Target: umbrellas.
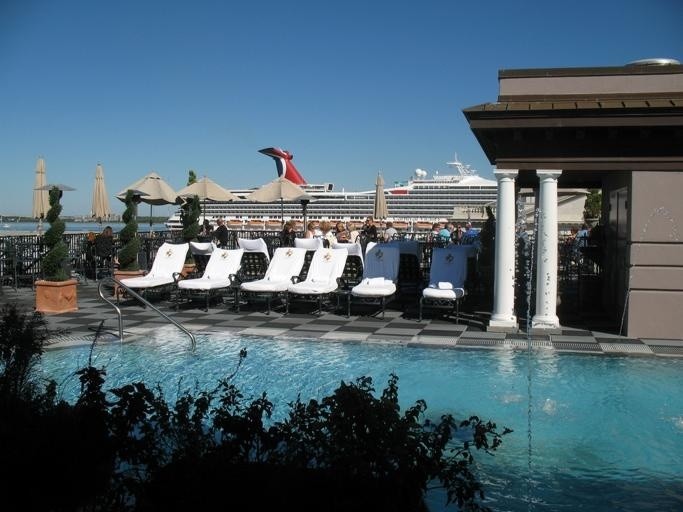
[91,159,112,233]
[114,168,389,234]
[32,153,51,235]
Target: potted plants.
[32,185,79,316]
[113,171,201,307]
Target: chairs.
[391,240,429,292]
[238,238,269,282]
[294,238,323,281]
[448,244,476,265]
[419,246,469,325]
[285,248,348,317]
[178,248,244,312]
[190,242,217,278]
[332,243,365,314]
[239,247,308,315]
[120,241,189,309]
[347,242,401,323]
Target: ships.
[164,145,499,236]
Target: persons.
[96,225,113,258]
[429,222,478,241]
[280,216,399,246]
[197,219,228,248]
